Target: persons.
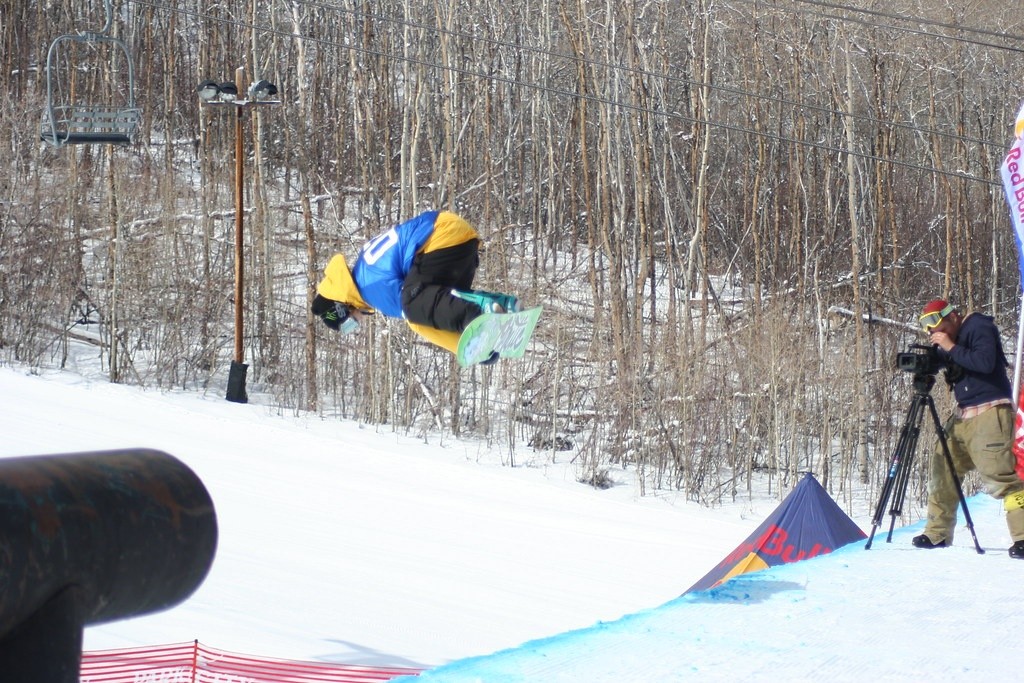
[310,209,519,367]
[911,299,1024,560]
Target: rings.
[938,333,940,336]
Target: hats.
[311,294,349,334]
[924,297,947,313]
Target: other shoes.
[479,302,504,365]
[1009,541,1024,558]
[913,535,945,548]
[506,297,525,313]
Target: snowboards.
[456,305,544,367]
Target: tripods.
[864,375,984,554]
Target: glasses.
[338,315,360,335]
[919,311,942,332]
